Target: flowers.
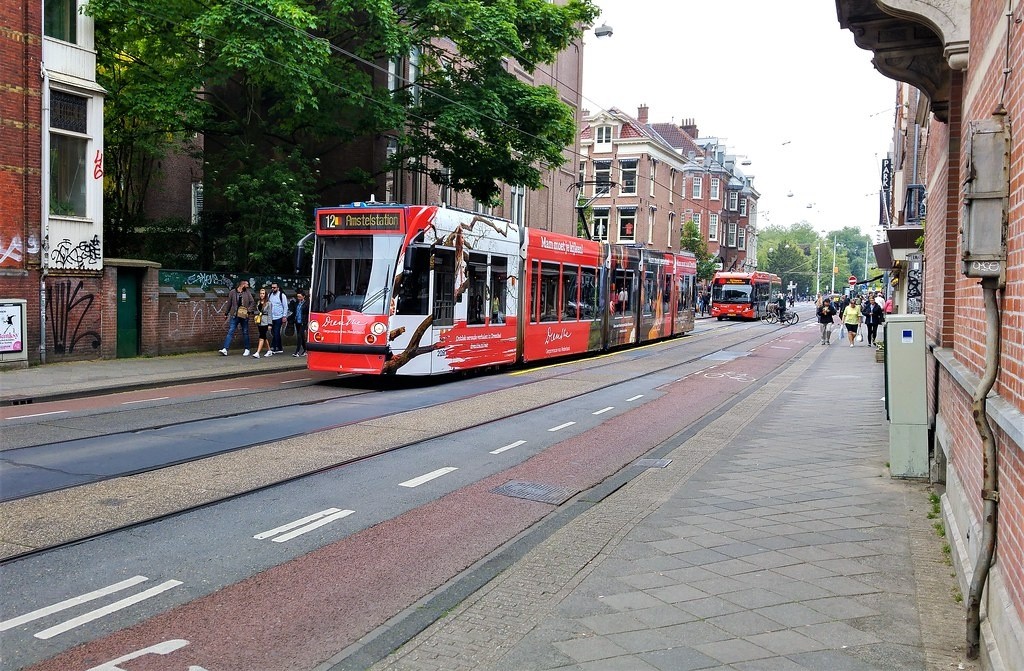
[873,339,884,350]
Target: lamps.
[821,228,826,233]
[785,243,790,248]
[805,203,813,208]
[786,192,794,197]
[740,158,752,165]
[835,242,842,247]
[768,244,774,252]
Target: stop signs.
[848,276,857,286]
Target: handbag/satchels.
[254,312,262,324]
[839,325,844,339]
[856,327,863,342]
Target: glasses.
[851,303,855,304]
[271,287,276,289]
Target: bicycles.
[760,304,800,326]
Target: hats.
[267,330,274,339]
[877,293,881,295]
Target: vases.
[875,348,884,363]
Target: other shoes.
[868,343,871,347]
[872,339,876,346]
[827,341,830,345]
[822,341,825,345]
[292,352,300,357]
[219,348,227,356]
[243,349,251,356]
[850,344,855,348]
[264,351,272,357]
[272,350,283,354]
[301,350,308,356]
[253,352,260,358]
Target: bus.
[708,271,783,321]
[304,181,699,378]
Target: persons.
[771,293,786,325]
[267,280,288,354]
[610,287,629,316]
[698,290,711,313]
[817,298,837,346]
[290,290,309,357]
[862,298,884,347]
[252,287,274,358]
[813,290,893,325]
[218,278,256,357]
[474,291,483,323]
[842,298,863,348]
[777,291,815,308]
[493,293,500,323]
